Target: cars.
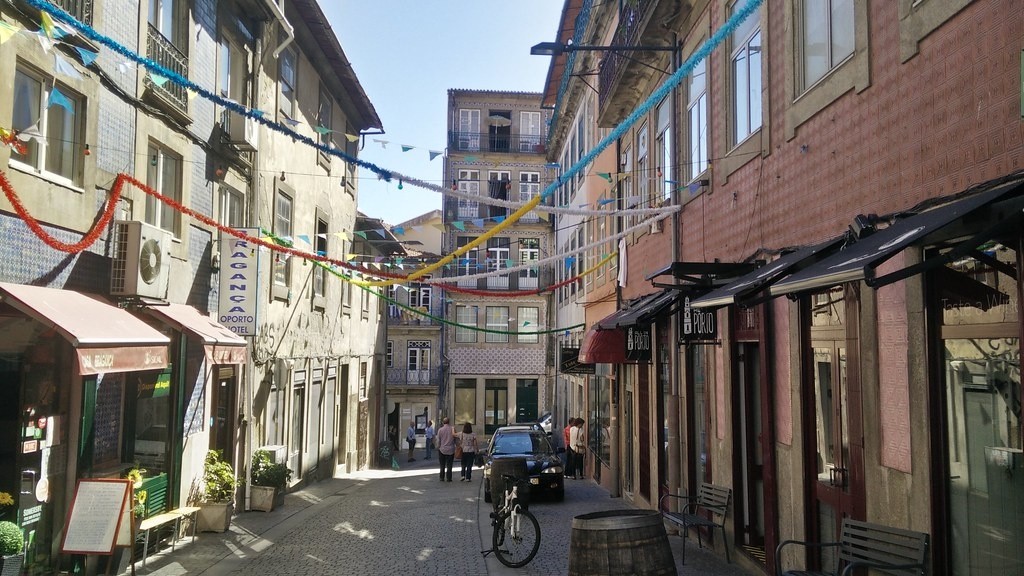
[507,412,552,437]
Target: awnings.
[0,283,171,377]
[688,234,856,309]
[645,260,767,299]
[140,301,249,365]
[590,290,710,333]
[578,308,660,364]
[761,170,1024,303]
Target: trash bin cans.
[378,440,393,468]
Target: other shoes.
[440,478,444,481]
[460,477,465,481]
[466,479,469,481]
[571,477,576,480]
[580,477,585,479]
[447,480,452,482]
[408,460,413,462]
[411,458,416,461]
[567,476,574,478]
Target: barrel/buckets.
[568,509,677,576]
[489,458,529,511]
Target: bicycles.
[480,472,541,569]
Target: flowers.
[0,491,14,508]
[123,459,148,519]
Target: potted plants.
[196,448,244,533]
[0,520,25,576]
[249,448,295,513]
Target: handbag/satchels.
[454,446,463,459]
[406,436,412,442]
[431,442,435,449]
[576,443,586,459]
[475,453,484,467]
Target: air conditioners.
[109,217,175,299]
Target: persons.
[406,421,416,462]
[425,421,434,460]
[564,417,576,478]
[452,422,479,482]
[569,418,586,480]
[389,423,399,451]
[437,418,459,482]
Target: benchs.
[775,510,930,576]
[659,481,731,564]
[92,457,136,478]
[138,506,201,567]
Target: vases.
[98,516,142,576]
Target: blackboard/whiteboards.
[58,477,134,555]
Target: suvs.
[482,426,565,502]
